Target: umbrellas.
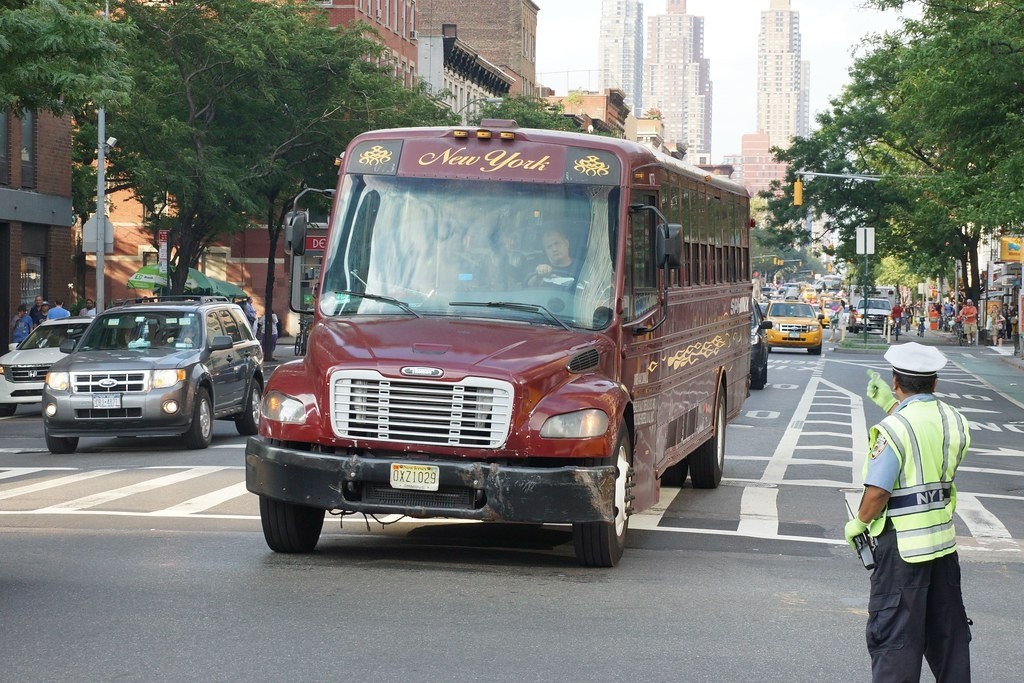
[126,263,249,300]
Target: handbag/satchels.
[997,323,1003,330]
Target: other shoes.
[967,341,975,347]
[991,343,997,346]
[838,340,845,342]
[827,339,834,343]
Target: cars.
[750,282,851,390]
[0,315,190,417]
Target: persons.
[258,310,278,361]
[890,299,1019,347]
[167,325,196,349]
[829,301,846,342]
[245,296,256,329]
[845,342,972,683]
[524,225,584,291]
[78,299,97,317]
[10,296,70,348]
[117,325,132,350]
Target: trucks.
[849,285,896,335]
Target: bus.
[245,119,756,569]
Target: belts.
[883,519,896,532]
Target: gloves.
[867,369,898,413]
[845,511,868,550]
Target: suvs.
[42,295,265,454]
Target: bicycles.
[294,319,307,356]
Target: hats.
[967,299,972,302]
[40,301,49,305]
[884,342,948,376]
